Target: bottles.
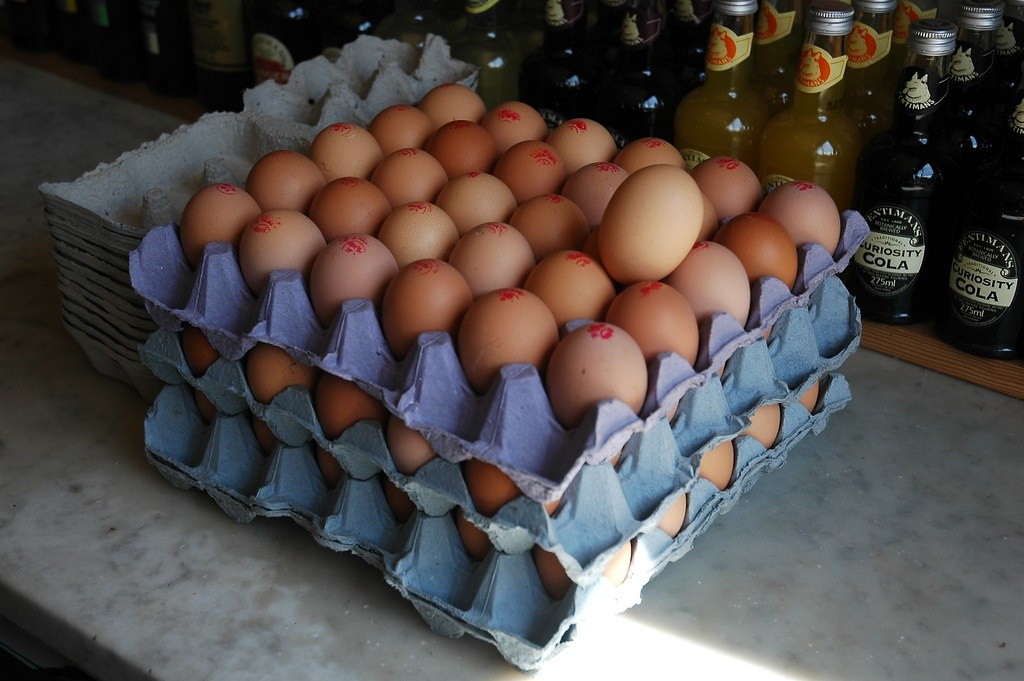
[892,0,939,69]
[844,0,899,142]
[852,19,959,326]
[751,0,805,120]
[0,0,713,184]
[758,0,861,220]
[676,0,769,171]
[931,0,1024,359]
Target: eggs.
[177,82,840,594]
